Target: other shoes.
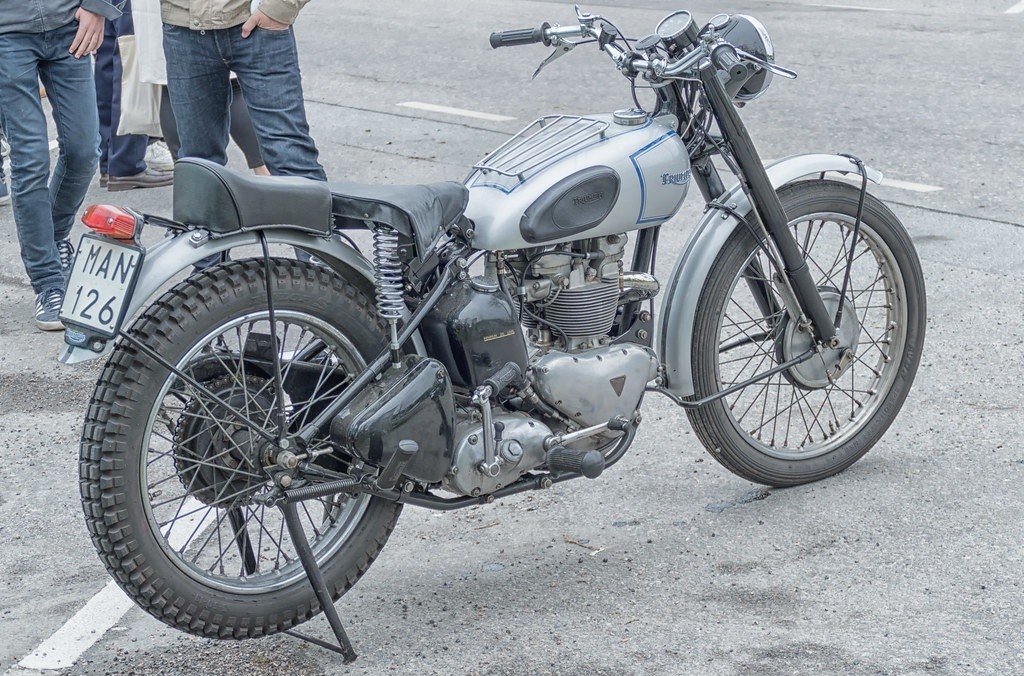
[0,178,10,203]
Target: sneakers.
[55,236,74,276]
[34,287,65,330]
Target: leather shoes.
[107,167,173,191]
[99,174,108,186]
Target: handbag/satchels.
[116,34,165,138]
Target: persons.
[0,0,127,332]
[131,0,340,278]
[94,0,174,192]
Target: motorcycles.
[58,3,928,662]
